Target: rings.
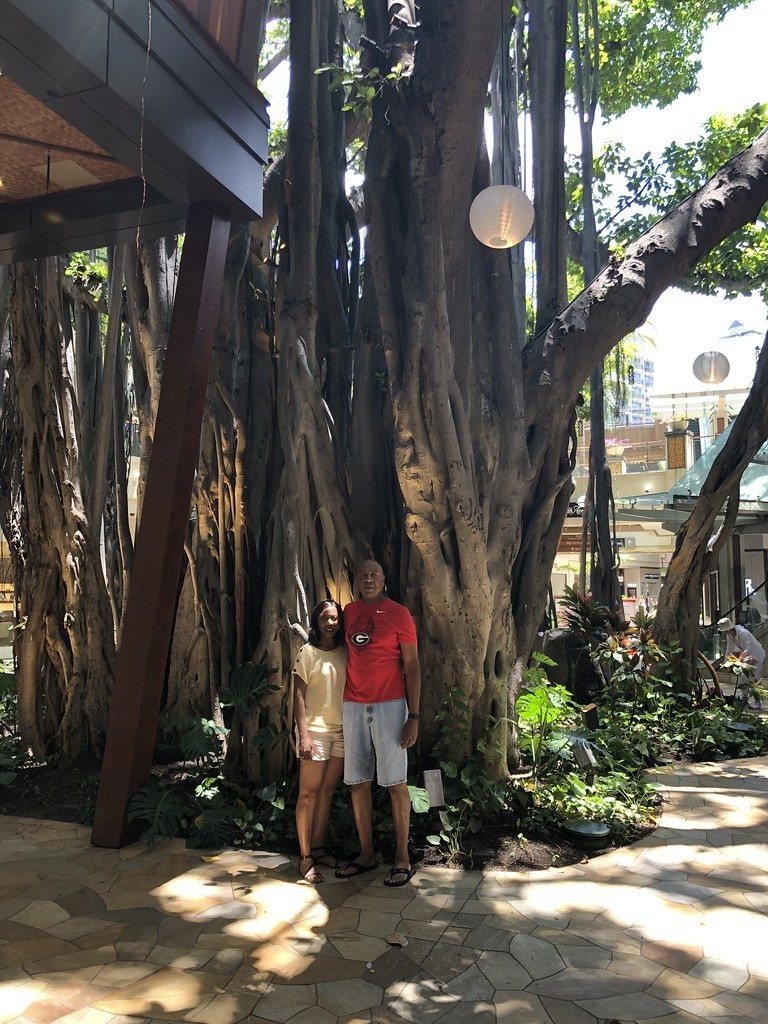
[412,742,416,744]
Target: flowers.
[605,437,632,448]
[659,413,697,426]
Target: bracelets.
[408,714,419,720]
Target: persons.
[335,560,420,886]
[291,599,349,884]
[717,619,766,709]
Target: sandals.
[311,847,349,869]
[299,854,324,883]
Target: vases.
[607,447,625,458]
[671,420,690,430]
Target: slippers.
[384,864,416,887]
[335,858,378,878]
